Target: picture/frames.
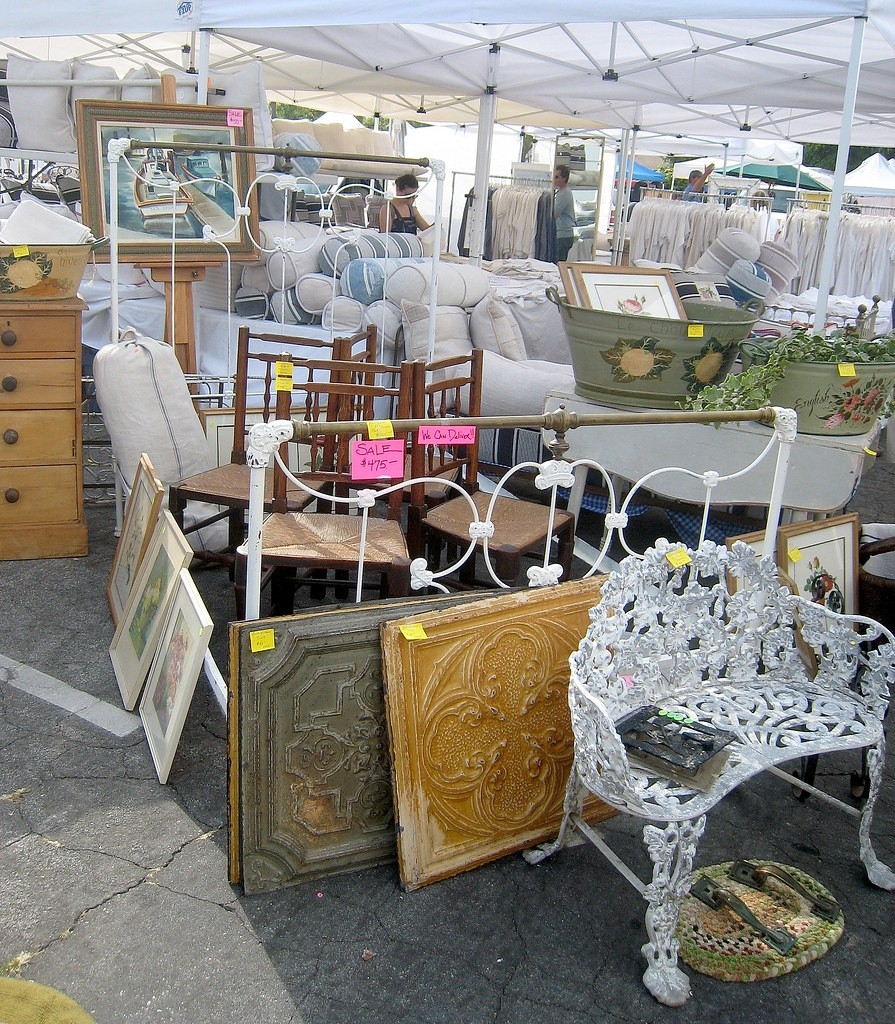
[139,567,214,785]
[571,265,688,321]
[725,520,814,709]
[75,98,262,264]
[107,452,165,629]
[777,511,860,671]
[108,509,194,711]
[557,261,656,307]
[200,405,371,529]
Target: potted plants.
[674,323,895,436]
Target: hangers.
[791,199,895,223]
[730,195,769,213]
[489,174,551,193]
[643,187,727,211]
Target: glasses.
[555,175,561,179]
[401,190,418,199]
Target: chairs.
[335,323,462,602]
[412,347,577,596]
[522,537,895,1008]
[234,350,414,619]
[169,325,342,624]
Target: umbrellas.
[716,162,833,191]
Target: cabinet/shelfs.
[0,290,90,561]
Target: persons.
[379,175,430,236]
[553,165,576,262]
[685,163,715,205]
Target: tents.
[615,154,760,191]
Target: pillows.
[266,229,378,291]
[567,170,582,187]
[276,118,395,226]
[321,295,366,332]
[755,240,800,308]
[0,53,275,217]
[662,267,737,310]
[235,288,272,320]
[240,264,275,296]
[270,291,321,326]
[236,222,326,266]
[400,299,474,413]
[382,264,489,308]
[317,233,424,279]
[725,260,772,313]
[684,227,760,274]
[631,259,682,272]
[296,273,341,315]
[469,291,528,361]
[453,349,578,429]
[339,257,438,305]
[363,300,406,349]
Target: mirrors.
[552,135,606,261]
